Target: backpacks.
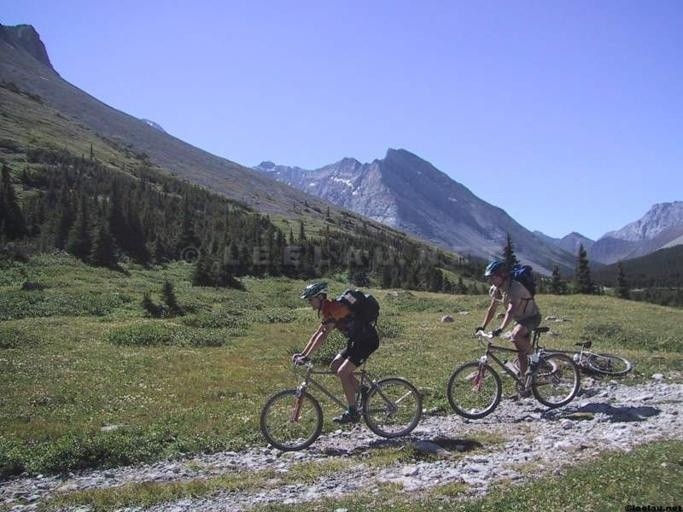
[333,287,379,324]
[507,264,535,301]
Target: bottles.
[573,354,578,362]
[502,358,520,376]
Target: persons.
[472,260,542,401]
[291,280,380,424]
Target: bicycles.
[445,324,583,420]
[510,340,634,377]
[257,354,424,454]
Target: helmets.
[300,282,327,300]
[483,259,505,278]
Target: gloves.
[292,354,305,363]
[492,328,501,336]
[295,356,307,366]
[476,326,483,332]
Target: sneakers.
[360,386,370,407]
[509,390,532,399]
[524,354,540,376]
[331,411,360,424]
